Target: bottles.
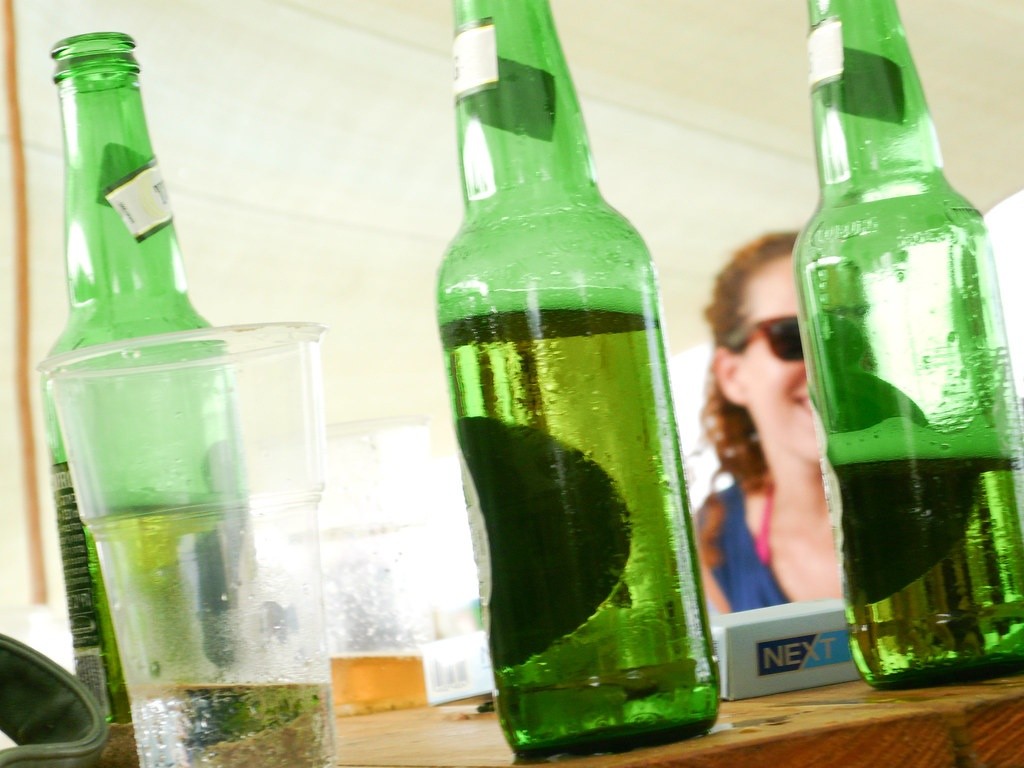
[790,2,1023,696]
[38,32,288,768]
[434,1,721,764]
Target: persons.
[695,231,844,614]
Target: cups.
[40,321,335,766]
[314,412,443,719]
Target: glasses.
[728,316,806,362]
